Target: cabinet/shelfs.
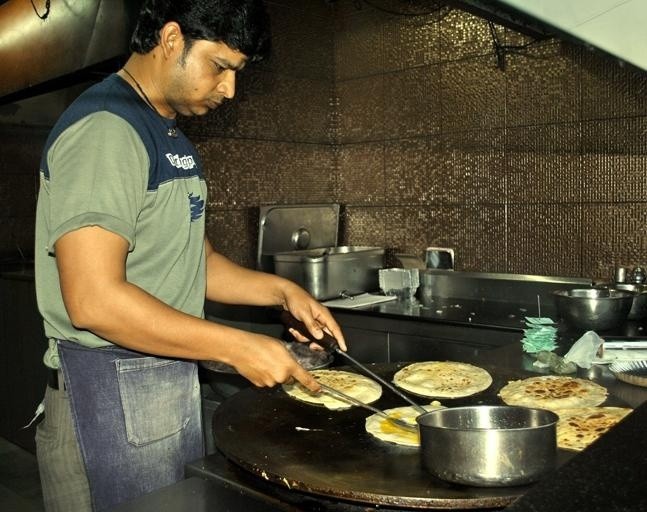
[328,289,647,364]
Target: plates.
[608,360,647,388]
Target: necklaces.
[122,67,178,138]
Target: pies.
[547,408,606,450]
[280,369,381,411]
[394,361,493,399]
[365,400,448,447]
[499,375,607,408]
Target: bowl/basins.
[199,342,334,399]
[554,288,640,330]
[594,283,647,318]
[415,405,559,487]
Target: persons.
[22,0,347,512]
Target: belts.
[47,366,66,391]
